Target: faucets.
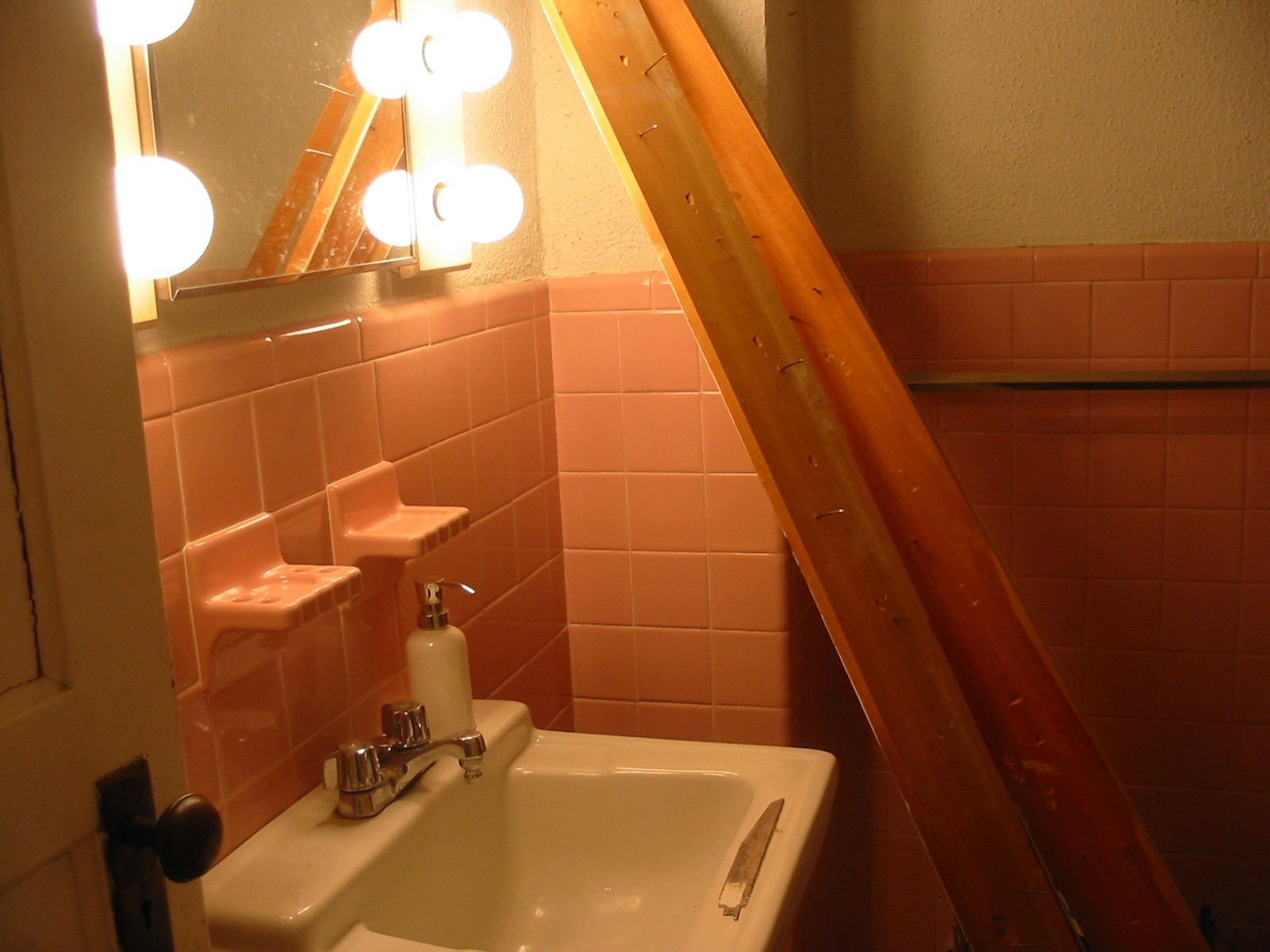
[337,698,487,823]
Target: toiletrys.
[405,574,479,738]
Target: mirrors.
[130,0,418,302]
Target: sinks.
[201,697,845,951]
